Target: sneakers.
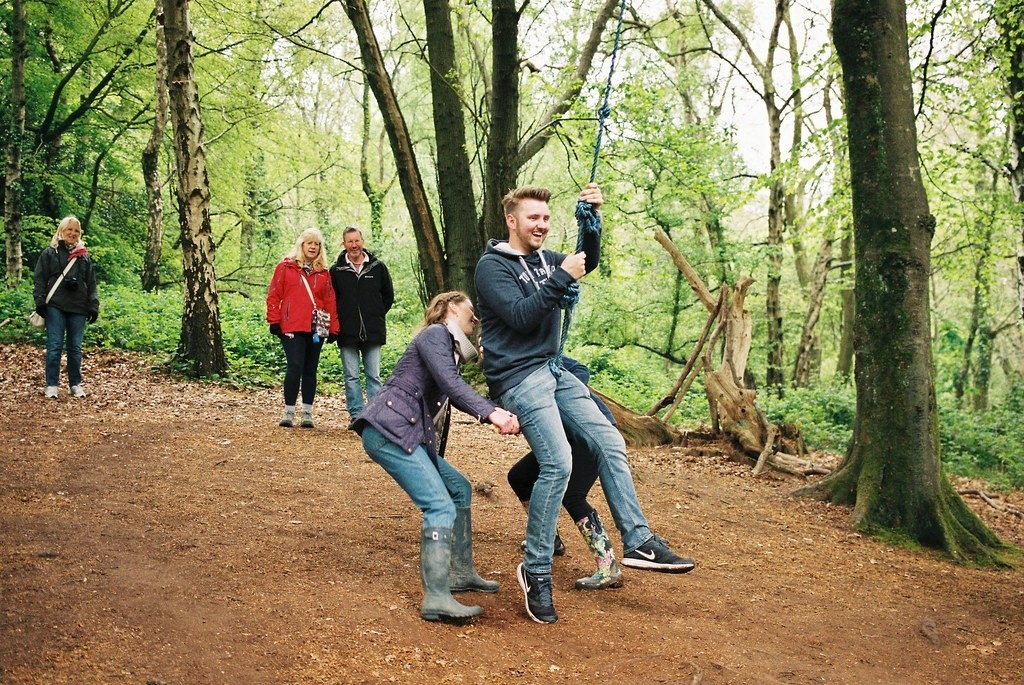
[620,533,695,574]
[517,562,559,624]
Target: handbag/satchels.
[311,308,330,336]
[29,310,45,329]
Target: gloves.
[36,304,47,317]
[270,322,281,334]
[326,333,337,343]
[70,384,86,397]
[87,310,98,323]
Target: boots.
[521,500,566,555]
[420,526,483,620]
[448,507,499,592]
[575,508,623,590]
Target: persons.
[329,226,394,431]
[348,291,515,624]
[477,326,626,591]
[32,216,101,400]
[473,181,695,624]
[267,227,340,429]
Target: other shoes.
[300,411,314,427]
[45,385,58,398]
[279,410,295,426]
